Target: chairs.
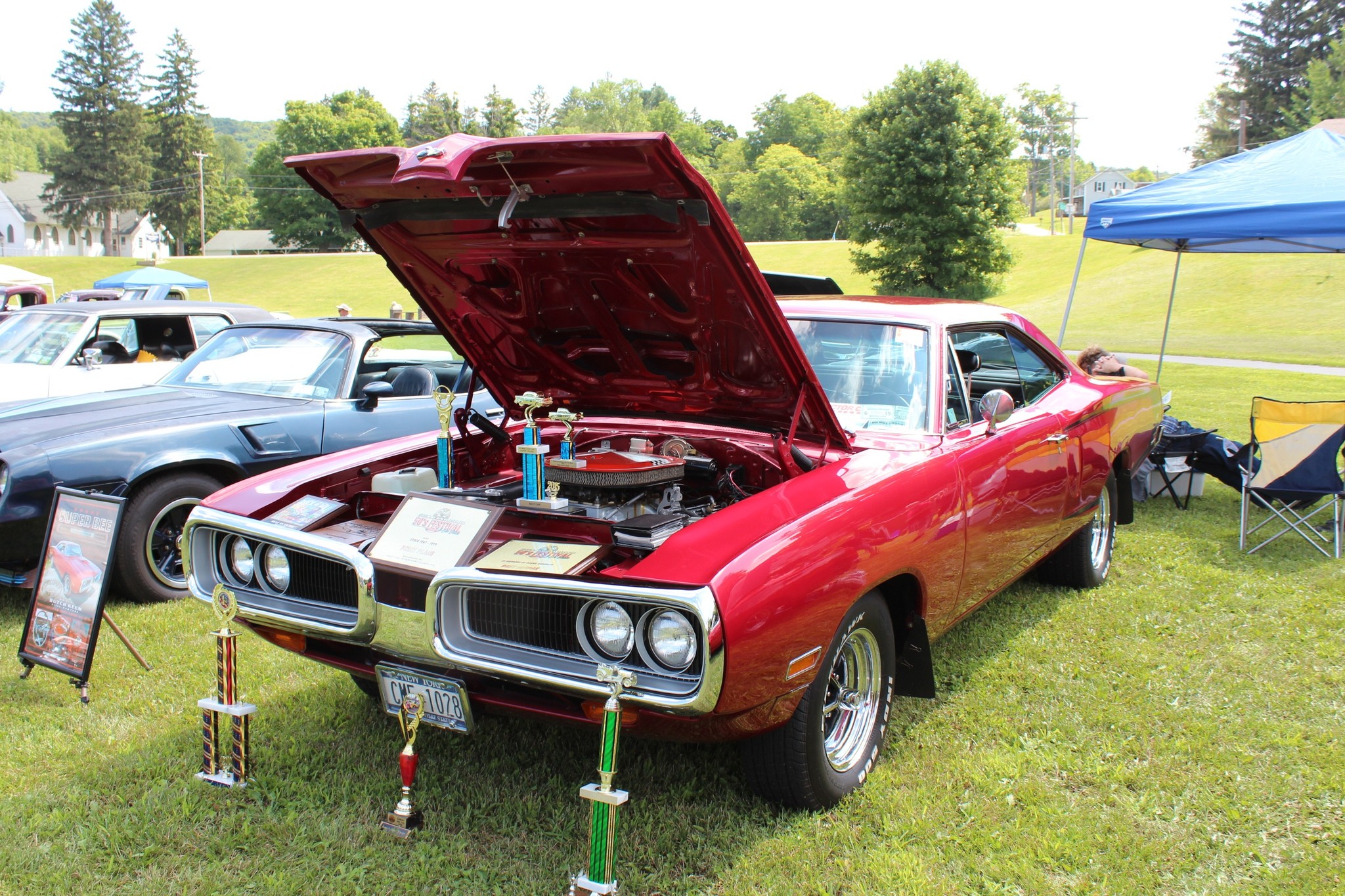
[947,348,986,424]
[389,366,440,400]
[1148,406,1219,511]
[135,337,180,363]
[92,340,130,365]
[1223,397,1345,558]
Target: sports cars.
[0,318,505,602]
[0,301,452,430]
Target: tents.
[1058,126,1345,382]
[0,264,213,304]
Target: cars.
[0,284,189,323]
[181,132,1164,896]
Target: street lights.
[1062,103,1088,235]
[1038,122,1065,235]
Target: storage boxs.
[1146,464,1206,496]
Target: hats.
[337,304,352,311]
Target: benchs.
[358,368,482,396]
[823,373,1039,410]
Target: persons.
[1075,346,1323,512]
[337,303,352,317]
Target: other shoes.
[1286,497,1322,509]
